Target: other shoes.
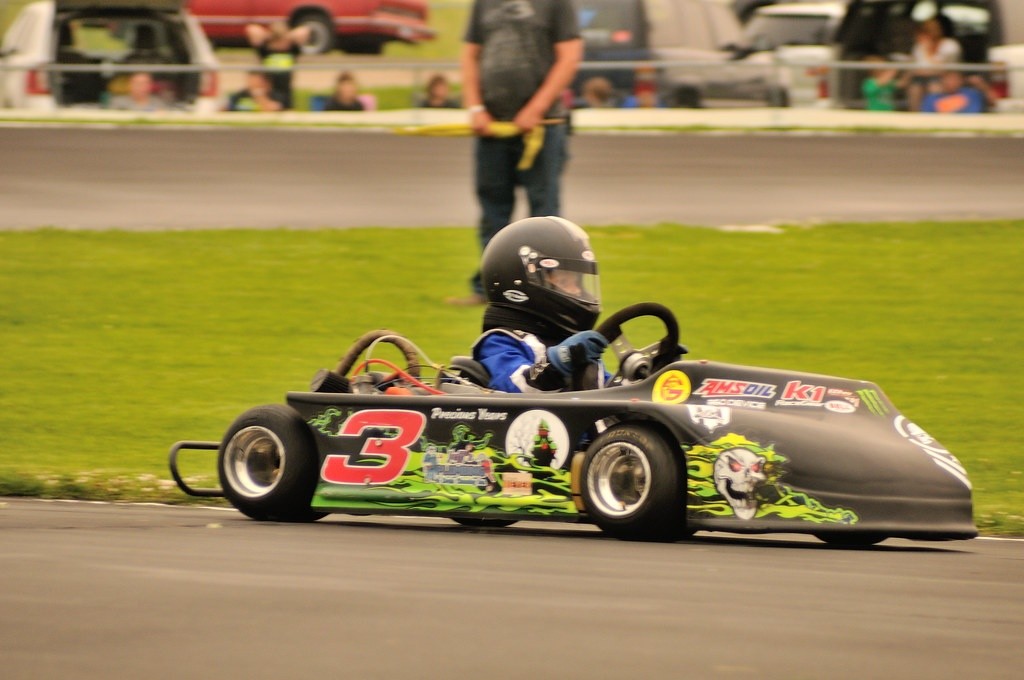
[444,294,485,307]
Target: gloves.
[652,344,688,373]
[547,330,608,377]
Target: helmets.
[480,215,600,334]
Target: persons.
[109,23,704,109]
[862,15,984,113]
[449,0,584,307]
[472,216,688,393]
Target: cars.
[558,0,1023,119]
[185,0,437,57]
[1,0,232,121]
